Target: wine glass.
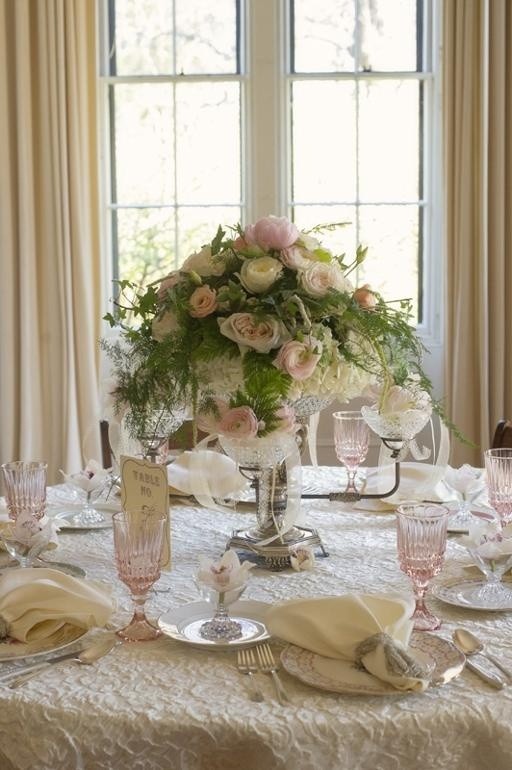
[332,412,371,493]
[484,448,511,541]
[467,545,511,599]
[441,476,487,521]
[64,474,108,520]
[0,526,49,568]
[395,503,447,629]
[193,579,247,637]
[1,460,47,522]
[112,513,166,643]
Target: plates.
[45,503,120,529]
[1,562,86,582]
[431,576,511,612]
[156,597,276,651]
[446,509,496,534]
[0,621,90,662]
[281,629,464,695]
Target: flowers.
[96,214,478,503]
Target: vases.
[124,392,434,571]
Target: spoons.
[455,628,512,685]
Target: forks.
[10,637,116,689]
[255,644,288,707]
[234,648,265,704]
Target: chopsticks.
[1,641,124,680]
